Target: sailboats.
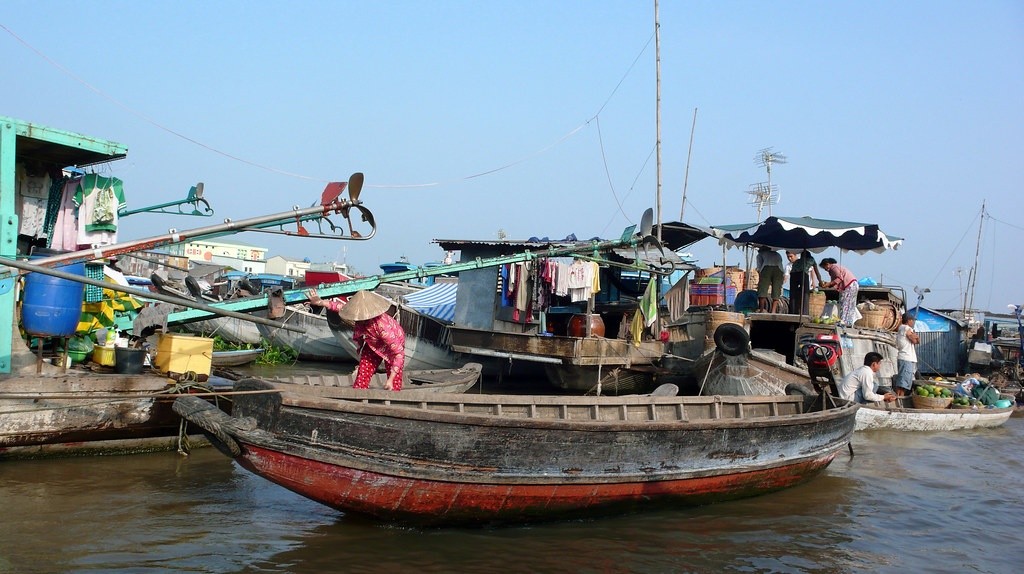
[168,0,861,521]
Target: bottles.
[105,326,115,347]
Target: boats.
[1,117,486,450]
[677,149,1024,435]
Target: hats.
[902,313,917,321]
[758,246,771,253]
[338,291,391,321]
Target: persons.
[304,289,405,391]
[784,251,816,297]
[839,352,896,405]
[977,323,985,342]
[790,250,823,314]
[895,313,920,397]
[756,246,783,313]
[820,258,859,325]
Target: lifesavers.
[171,394,257,459]
[185,276,202,298]
[714,322,750,358]
[241,280,259,295]
[152,274,164,290]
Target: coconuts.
[915,385,982,406]
[813,315,840,325]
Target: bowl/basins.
[56,349,88,361]
[996,399,1011,408]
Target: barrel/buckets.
[56,353,72,369]
[22,247,85,338]
[114,344,148,374]
[43,358,51,362]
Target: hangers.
[80,162,115,181]
[575,247,594,263]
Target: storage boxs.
[94,344,117,366]
[688,269,736,306]
[155,333,214,377]
